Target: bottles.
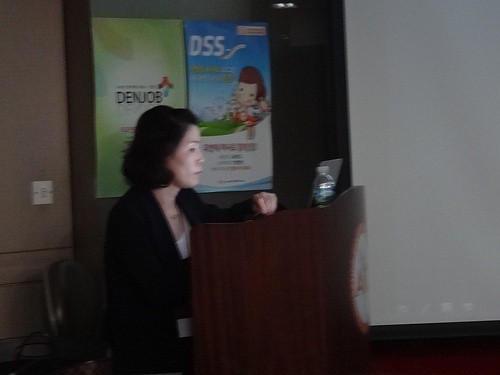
[312,166,337,208]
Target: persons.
[98,105,277,375]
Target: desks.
[0,336,113,375]
